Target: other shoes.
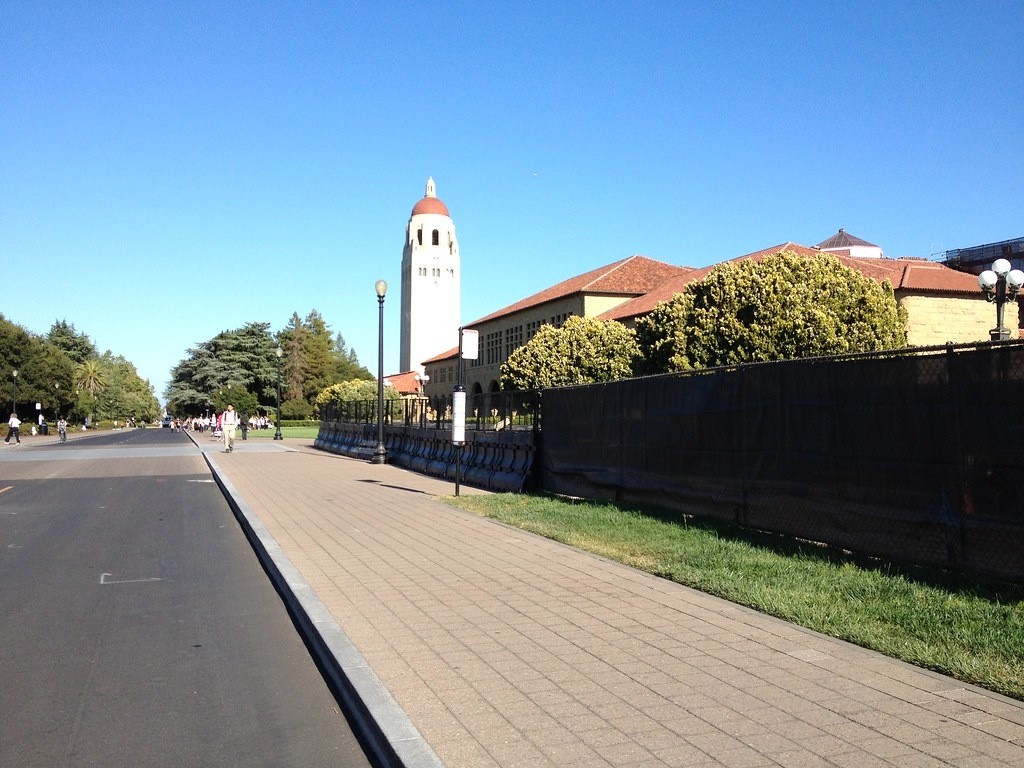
[17,442,19,444]
[243,437,247,440]
[226,449,229,453]
[230,447,233,451]
[4,441,10,445]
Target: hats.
[243,412,246,414]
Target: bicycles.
[58,427,66,445]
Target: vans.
[162,415,173,428]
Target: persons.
[141,421,145,428]
[58,418,67,441]
[175,419,181,432]
[240,412,249,440]
[114,420,117,429]
[3,413,21,445]
[183,417,210,433]
[218,412,223,431]
[220,403,238,452]
[249,416,274,430]
[210,412,218,436]
[169,420,176,433]
[120,422,124,429]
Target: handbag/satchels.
[213,427,221,437]
[240,421,246,425]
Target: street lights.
[12,370,18,415]
[219,391,223,411]
[974,259,1023,340]
[54,383,61,419]
[273,345,284,440]
[226,383,232,407]
[370,279,388,465]
[75,389,80,428]
[412,374,430,399]
